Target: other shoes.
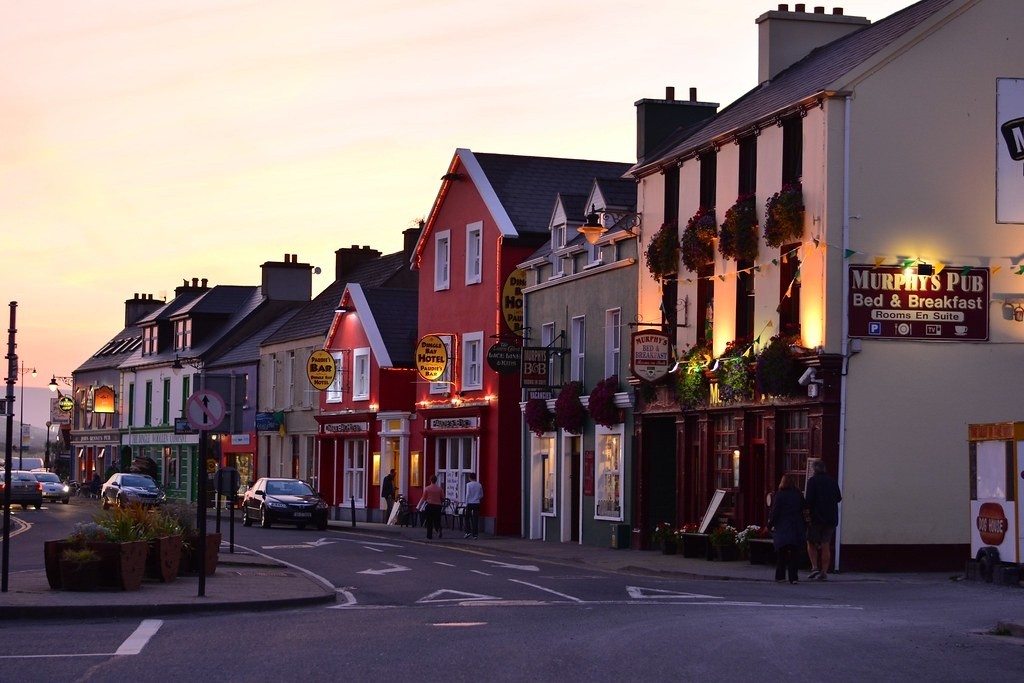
[438,528,442,538]
[425,538,430,542]
[775,578,786,583]
[808,569,820,578]
[473,536,477,540]
[791,580,798,585]
[818,572,826,579]
[464,533,471,539]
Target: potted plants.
[121,494,222,590]
[59,547,102,592]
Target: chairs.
[398,496,466,531]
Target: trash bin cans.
[610,523,630,549]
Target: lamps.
[48,374,76,393]
[169,354,203,376]
[669,357,732,372]
[577,203,641,245]
[917,257,932,275]
[334,306,350,313]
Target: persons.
[463,473,484,540]
[86,461,129,498]
[767,475,809,584]
[805,460,842,580]
[381,469,400,524]
[422,476,446,540]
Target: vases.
[659,540,678,555]
[679,531,708,557]
[748,537,775,564]
[45,539,120,592]
[716,543,741,562]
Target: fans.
[652,522,700,544]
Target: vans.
[11,456,44,472]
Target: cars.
[29,472,71,504]
[0,470,43,510]
[241,477,330,531]
[0,458,8,471]
[100,473,167,512]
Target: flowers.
[716,331,754,402]
[525,399,552,437]
[718,193,758,263]
[673,337,713,417]
[554,380,586,434]
[643,219,680,284]
[588,374,619,431]
[755,332,801,402]
[679,206,717,273]
[762,184,803,249]
[709,524,773,553]
[63,502,144,544]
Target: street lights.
[19,360,38,470]
